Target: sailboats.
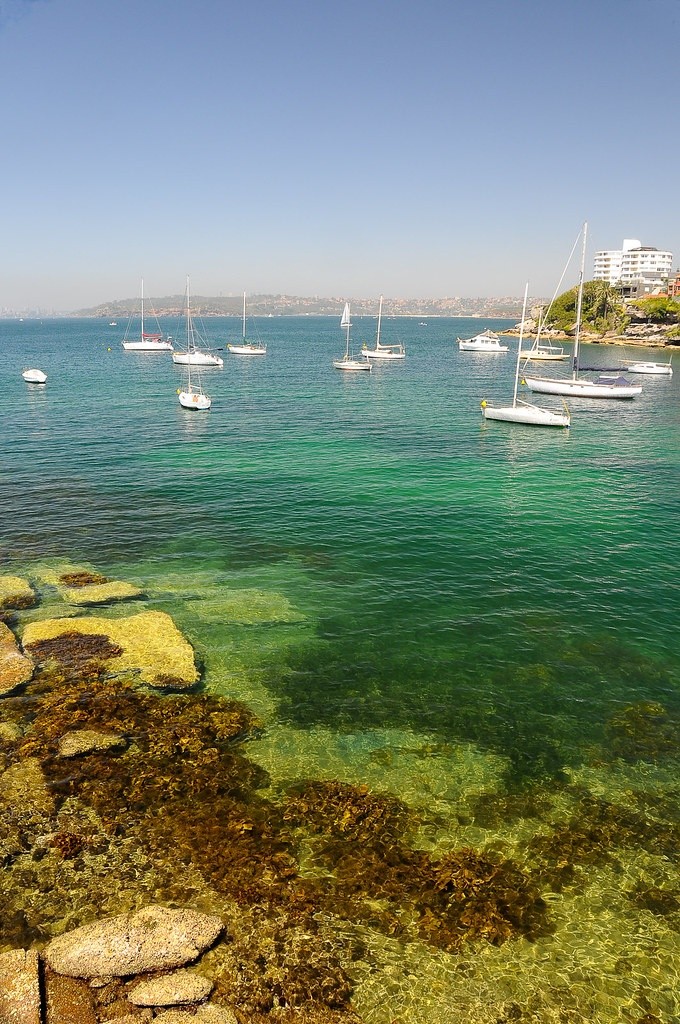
[517,309,571,360]
[480,280,572,428]
[120,275,267,411]
[331,294,406,371]
[513,220,645,399]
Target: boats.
[22,368,48,383]
[455,328,511,352]
[627,354,675,375]
